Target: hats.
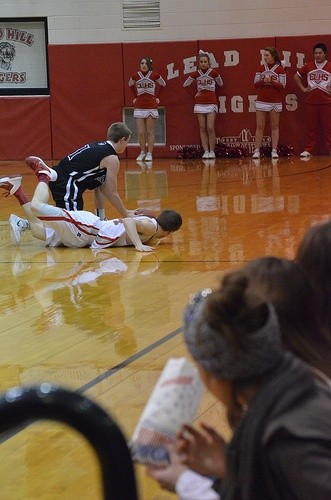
[181,279,281,377]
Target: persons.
[128,56,166,161]
[0,156,183,251]
[145,219,331,500]
[293,43,331,157]
[182,49,224,158]
[251,47,286,158]
[48,122,137,220]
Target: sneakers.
[300,150,311,157]
[0,174,22,198]
[24,155,59,181]
[8,214,30,245]
[252,149,260,159]
[271,150,279,159]
[201,151,216,160]
[134,152,154,161]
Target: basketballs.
[135,209,155,218]
